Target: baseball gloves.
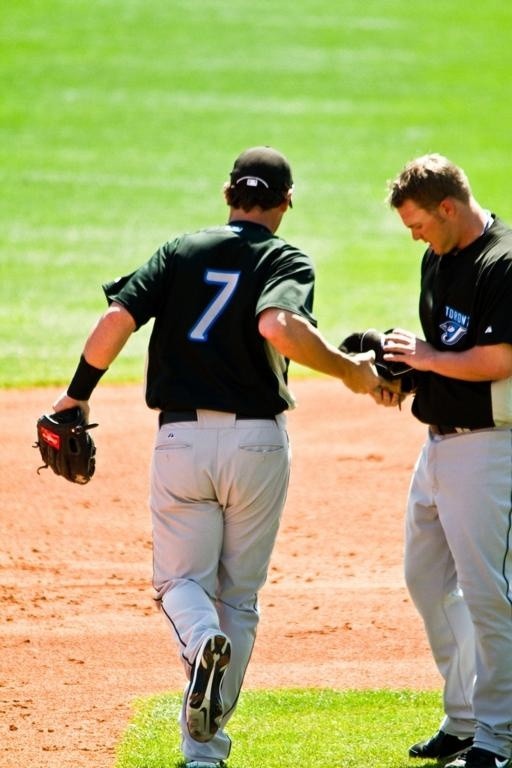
[31,406,98,484]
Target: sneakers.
[409,732,474,758]
[445,745,507,767]
[186,635,230,768]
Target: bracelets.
[65,357,106,402]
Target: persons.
[374,152,512,768]
[55,147,383,768]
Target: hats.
[229,145,294,209]
[338,329,422,393]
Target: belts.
[157,407,276,421]
[427,423,486,435]
